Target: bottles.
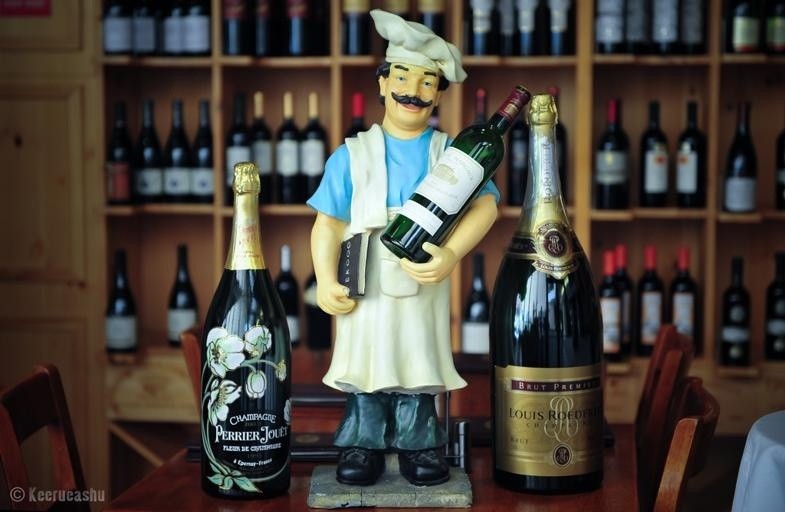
[593,98,785,212]
[466,0,577,56]
[343,93,368,144]
[106,88,331,204]
[489,92,608,497]
[273,244,303,348]
[598,242,785,369]
[196,160,294,502]
[340,0,446,57]
[724,1,785,53]
[595,1,707,55]
[104,1,332,57]
[163,241,203,346]
[377,75,531,266]
[106,242,138,365]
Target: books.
[335,231,372,299]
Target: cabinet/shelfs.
[103,0,785,512]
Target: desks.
[99,352,640,512]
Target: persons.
[305,6,500,487]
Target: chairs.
[180,323,205,413]
[634,322,719,512]
[0,361,93,512]
